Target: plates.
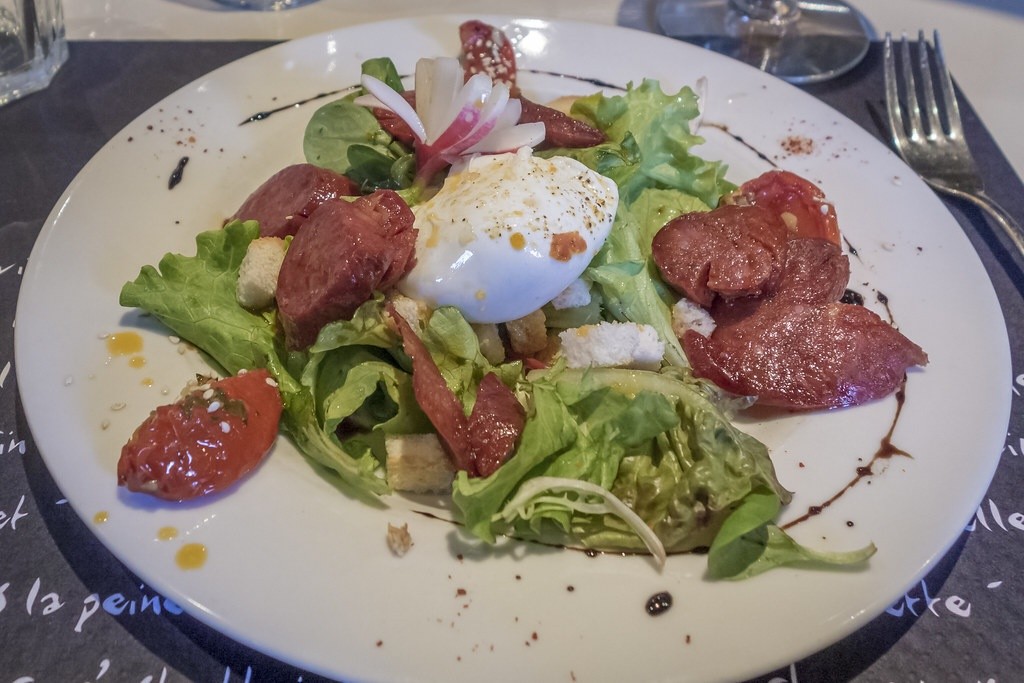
[14,15,1012,683]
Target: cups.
[0,0,69,109]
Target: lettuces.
[115,57,877,581]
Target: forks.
[884,27,1024,259]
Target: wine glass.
[654,0,871,84]
